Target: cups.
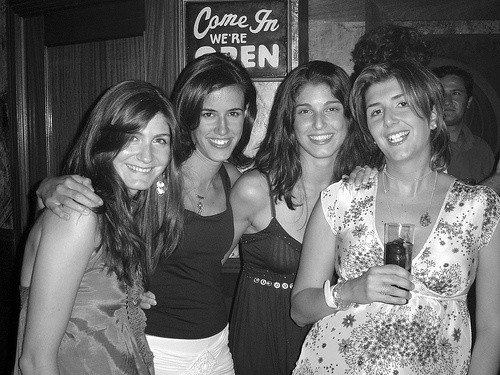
[384,222,415,305]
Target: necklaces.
[383,165,438,228]
[179,171,220,215]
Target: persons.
[36,52,258,375]
[290,57,500,375]
[0,79,185,375]
[220,61,380,375]
[434,67,497,185]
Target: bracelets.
[324,279,353,312]
